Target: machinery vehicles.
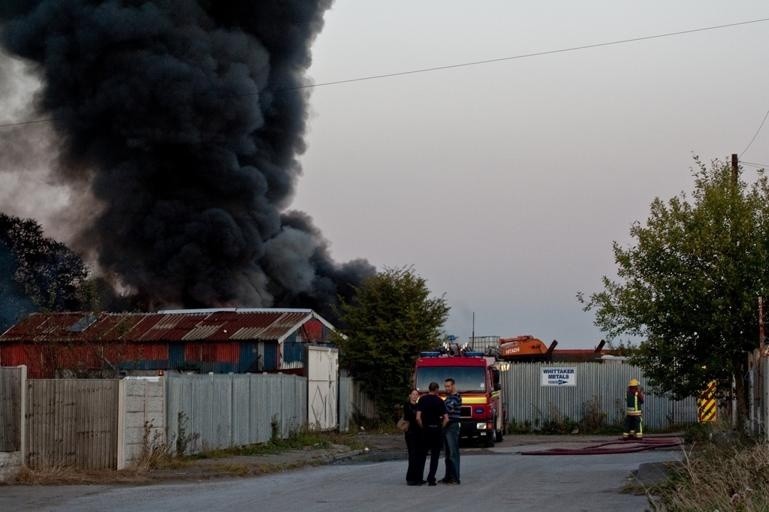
[481,334,549,354]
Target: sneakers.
[416,475,462,486]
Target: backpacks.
[397,418,410,433]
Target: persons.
[402,387,429,485]
[437,378,463,486]
[621,378,647,440]
[414,382,450,486]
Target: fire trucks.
[411,342,505,448]
